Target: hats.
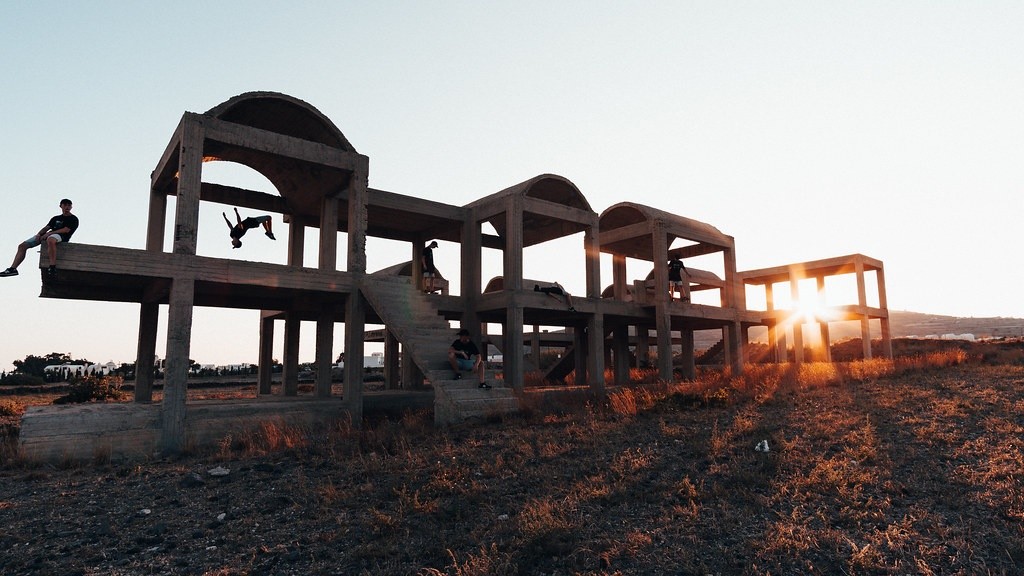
[534,285,539,291]
[432,241,438,248]
[61,199,72,205]
[457,329,469,336]
[233,242,241,248]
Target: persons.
[533,284,576,311]
[0,199,79,277]
[448,329,491,390]
[668,252,692,302]
[420,241,439,293]
[223,207,277,249]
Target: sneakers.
[0,268,18,277]
[48,268,58,281]
[569,306,577,313]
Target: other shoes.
[453,374,463,380]
[265,232,276,240]
[430,291,438,294]
[478,384,492,390]
